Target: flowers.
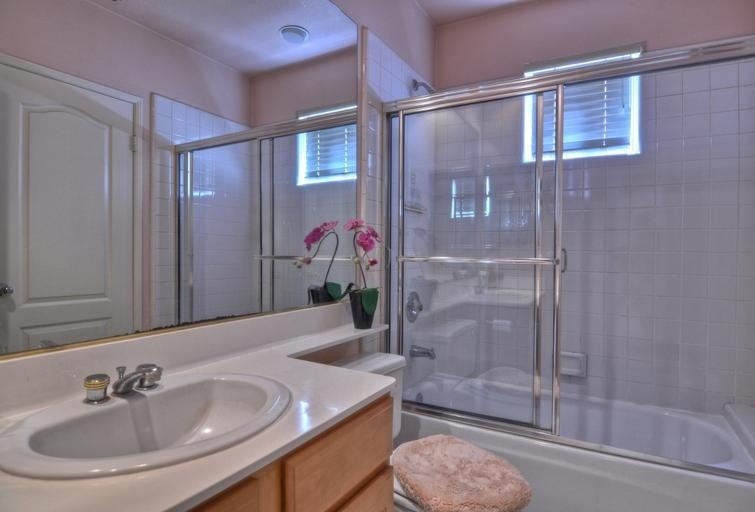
[292,219,342,300]
[344,215,383,315]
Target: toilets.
[335,353,518,510]
[430,320,549,390]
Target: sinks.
[1,370,292,477]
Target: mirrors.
[0,0,370,359]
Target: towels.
[391,432,533,512]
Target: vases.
[348,290,378,328]
[310,288,336,304]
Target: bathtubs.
[403,365,751,474]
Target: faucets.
[410,342,436,362]
[111,370,160,396]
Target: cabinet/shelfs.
[189,391,395,512]
